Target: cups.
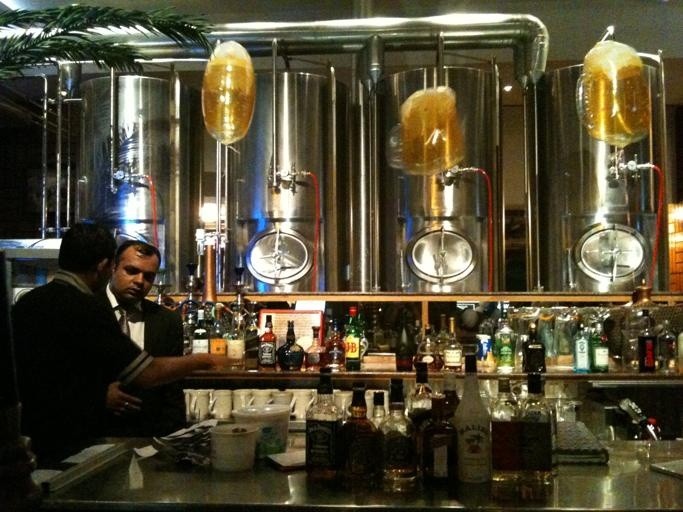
[179,386,355,420]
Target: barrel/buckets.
[232,402,289,456]
[211,423,258,471]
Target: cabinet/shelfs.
[141,287,682,386]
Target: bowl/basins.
[209,405,288,473]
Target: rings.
[121,400,129,408]
[119,406,125,411]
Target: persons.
[97,240,188,439]
[8,221,232,472]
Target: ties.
[118,308,135,338]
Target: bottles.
[304,361,582,502]
[186,302,678,375]
